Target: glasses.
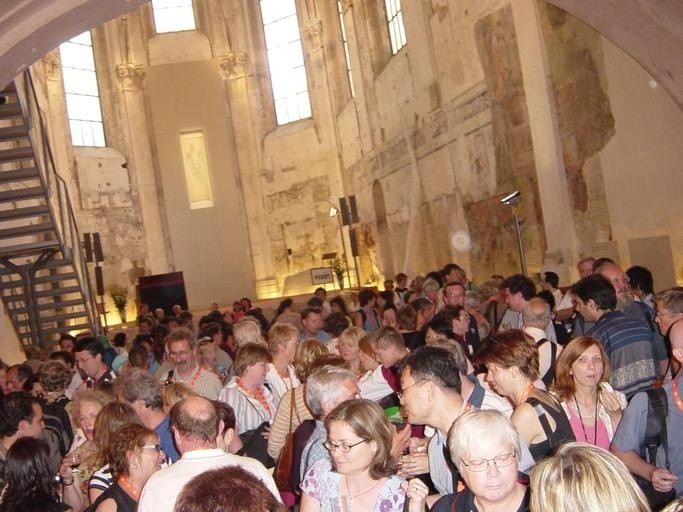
[322,438,372,455]
[168,350,193,360]
[140,444,163,454]
[395,378,431,401]
[460,447,519,473]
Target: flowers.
[328,257,346,279]
[110,288,131,310]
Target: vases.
[338,280,343,289]
[119,310,128,328]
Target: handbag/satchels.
[274,432,292,493]
[237,421,277,470]
[634,475,678,512]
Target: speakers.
[93,233,104,261]
[349,196,358,223]
[95,266,104,295]
[84,233,93,261]
[349,229,358,256]
[339,198,350,226]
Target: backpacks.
[37,394,75,477]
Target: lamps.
[330,207,351,289]
[501,190,528,279]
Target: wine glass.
[69,453,81,474]
[387,408,409,435]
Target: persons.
[1,258,682,512]
